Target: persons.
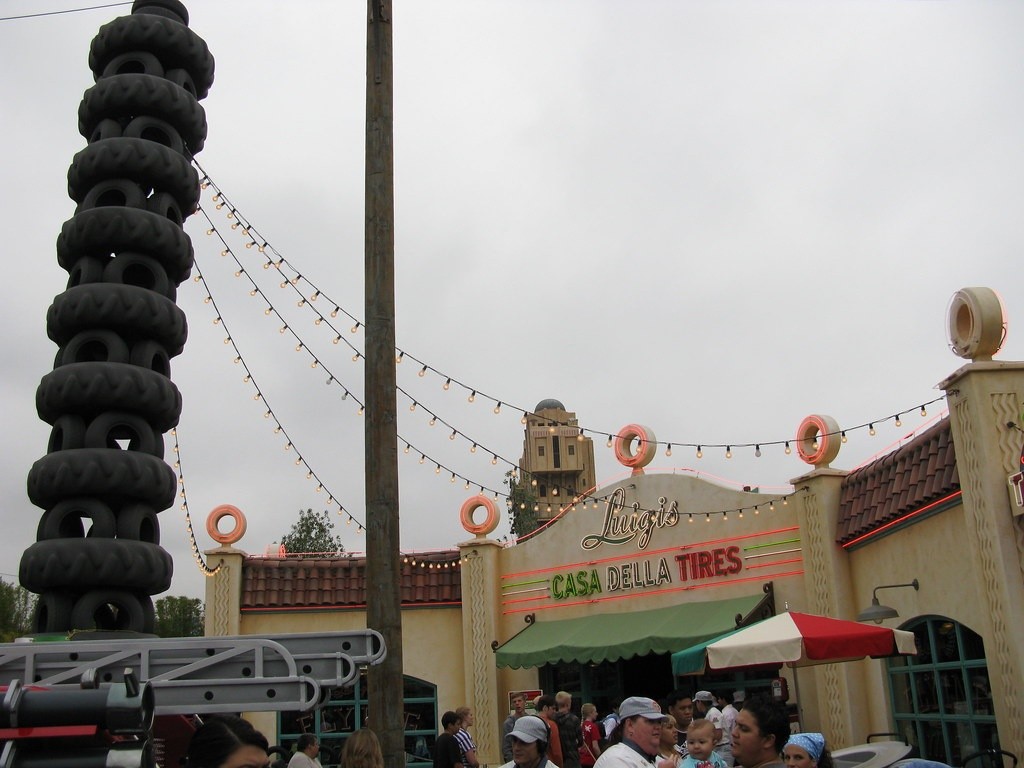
[187,715,268,768]
[287,733,323,768]
[496,691,834,768]
[432,707,479,768]
[341,728,383,768]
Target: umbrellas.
[673,601,918,734]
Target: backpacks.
[594,716,617,737]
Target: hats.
[533,696,542,704]
[619,696,670,723]
[505,715,550,743]
[692,691,713,702]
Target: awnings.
[491,581,775,670]
